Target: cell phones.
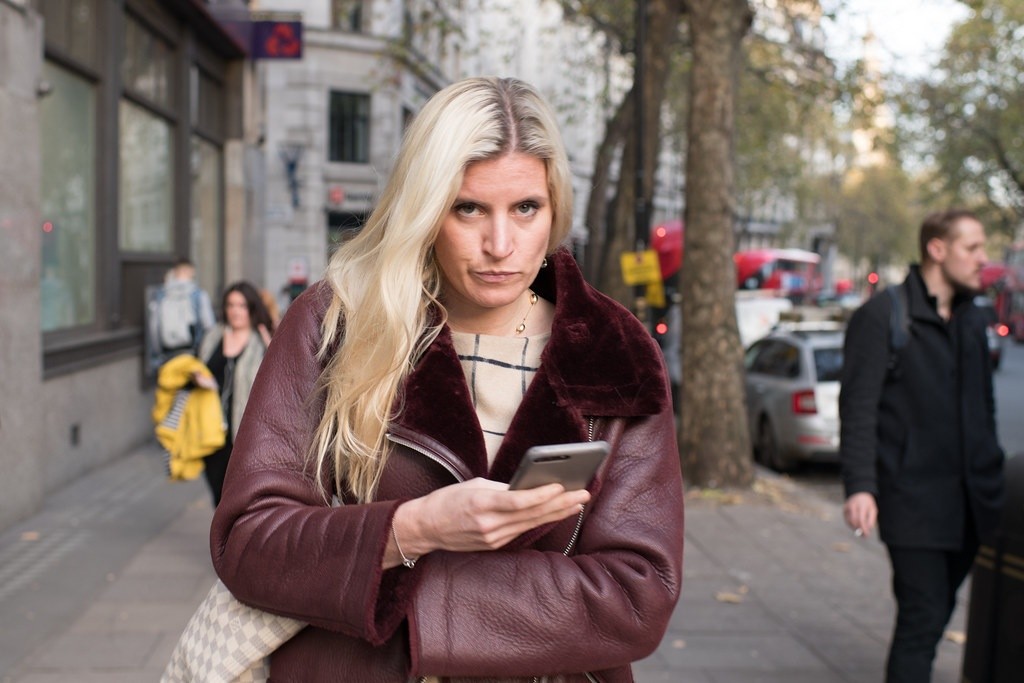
[507,441,610,491]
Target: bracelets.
[391,516,418,568]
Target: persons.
[209,77,683,683]
[145,260,214,371]
[838,209,1011,683]
[186,282,281,513]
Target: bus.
[733,249,822,305]
[980,241,1024,342]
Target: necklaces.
[502,286,540,339]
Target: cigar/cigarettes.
[853,528,864,539]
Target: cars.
[660,292,870,473]
[974,297,1000,369]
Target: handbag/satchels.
[158,495,347,683]
